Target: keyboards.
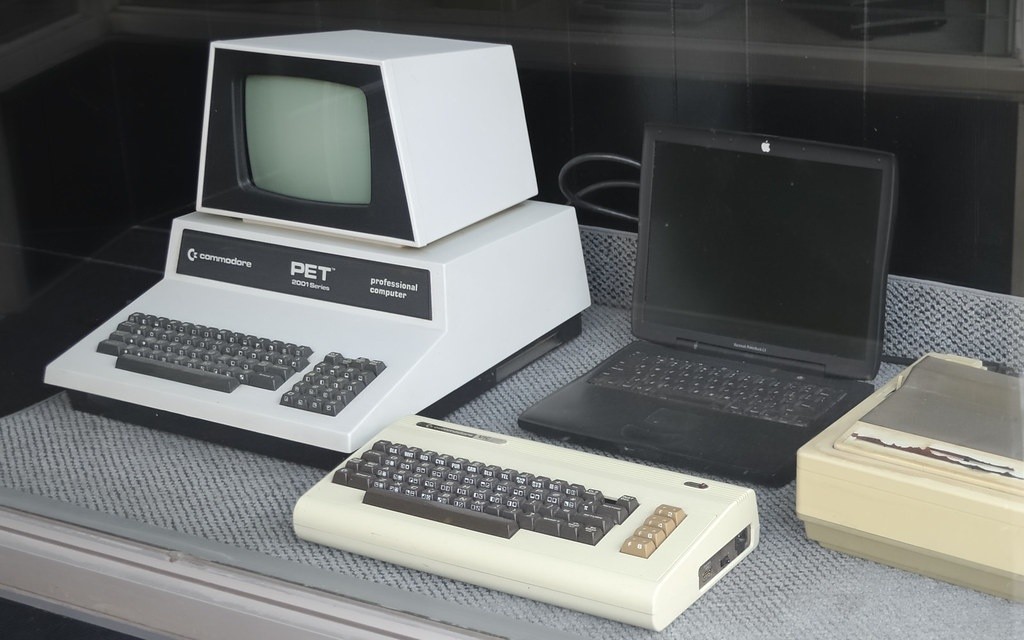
[290,413,765,633]
[95,310,389,417]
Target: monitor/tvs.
[195,28,539,249]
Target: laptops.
[513,121,902,491]
